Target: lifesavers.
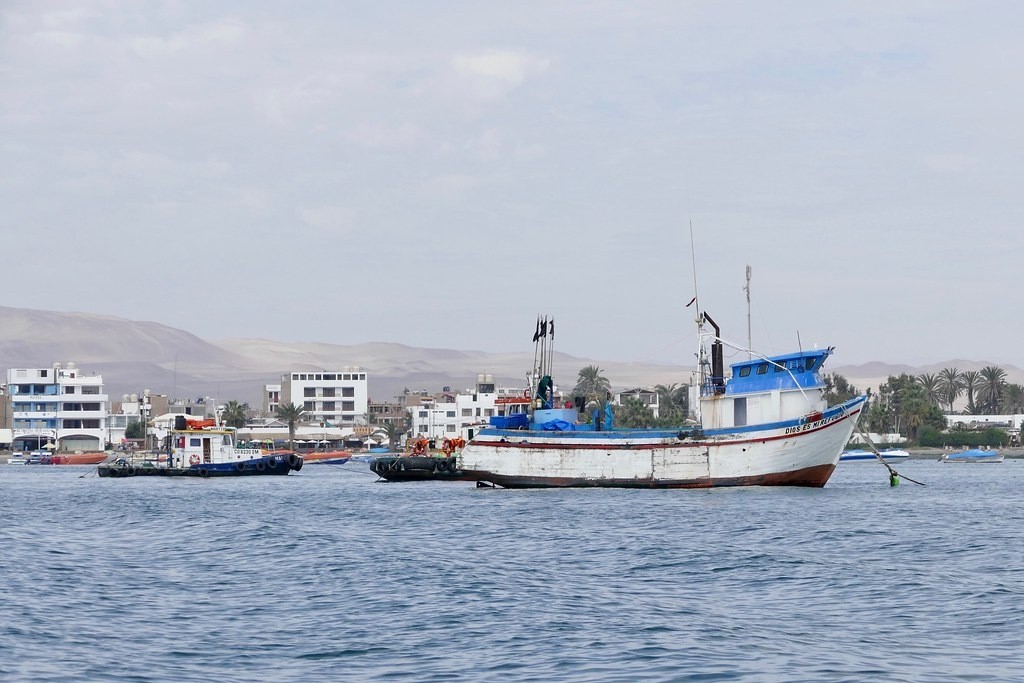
[390,459,401,472]
[378,460,388,474]
[447,456,459,472]
[236,462,245,471]
[267,458,278,468]
[287,454,298,467]
[198,468,208,476]
[256,462,266,471]
[188,453,200,465]
[126,467,136,476]
[437,460,448,471]
[145,468,152,475]
[294,457,303,471]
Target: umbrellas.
[284,439,330,448]
[251,439,274,449]
[148,413,204,431]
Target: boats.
[98,398,303,477]
[838,447,909,463]
[942,449,1004,463]
[370,457,485,481]
[261,450,351,465]
[457,218,868,489]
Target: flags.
[533,320,554,342]
